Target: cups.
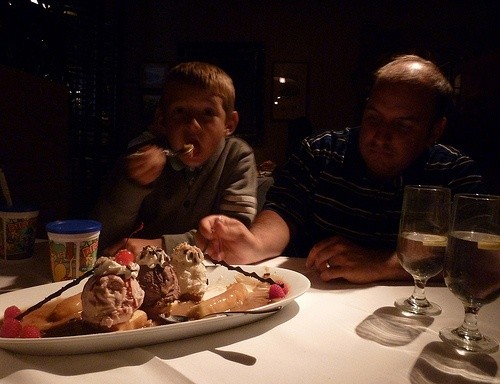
[0,211,40,260]
[46,219,102,282]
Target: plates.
[0,263,313,356]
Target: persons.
[190,50,492,285]
[88,62,260,255]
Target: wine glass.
[396,186,451,317]
[440,193,500,353]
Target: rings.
[326,259,330,271]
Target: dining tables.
[0,239,500,384]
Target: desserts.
[80,241,210,332]
